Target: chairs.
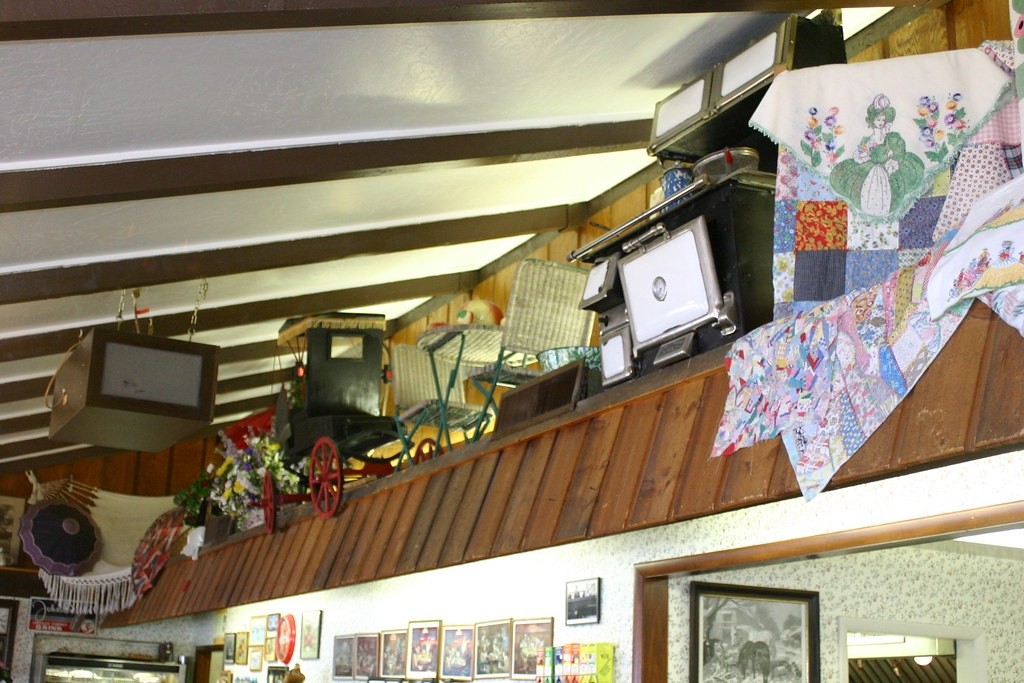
[467,258,597,446]
[390,344,496,475]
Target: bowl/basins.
[691,147,759,183]
[536,346,603,374]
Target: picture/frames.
[223,613,281,672]
[300,609,322,660]
[266,666,289,683]
[565,577,600,625]
[332,616,554,681]
[688,580,821,683]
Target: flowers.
[208,416,307,532]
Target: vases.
[246,508,265,529]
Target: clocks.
[275,613,296,664]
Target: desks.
[415,324,539,460]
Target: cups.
[658,160,692,199]
